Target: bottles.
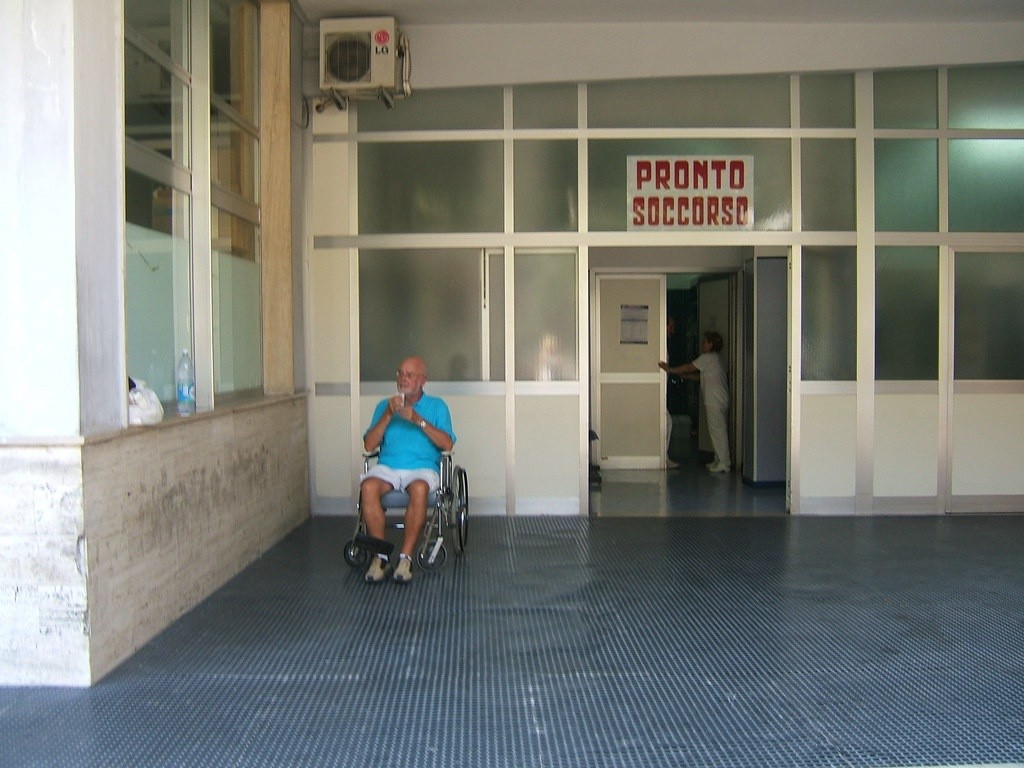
[177,349,195,417]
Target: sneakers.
[365,556,391,583]
[393,555,413,583]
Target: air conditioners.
[317,15,404,93]
[139,24,222,99]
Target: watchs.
[420,419,427,429]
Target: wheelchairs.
[344,448,470,574]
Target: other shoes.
[710,463,731,472]
[706,461,718,469]
[667,459,680,469]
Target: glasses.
[396,370,422,380]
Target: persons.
[658,315,731,472]
[364,357,457,583]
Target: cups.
[394,392,405,410]
